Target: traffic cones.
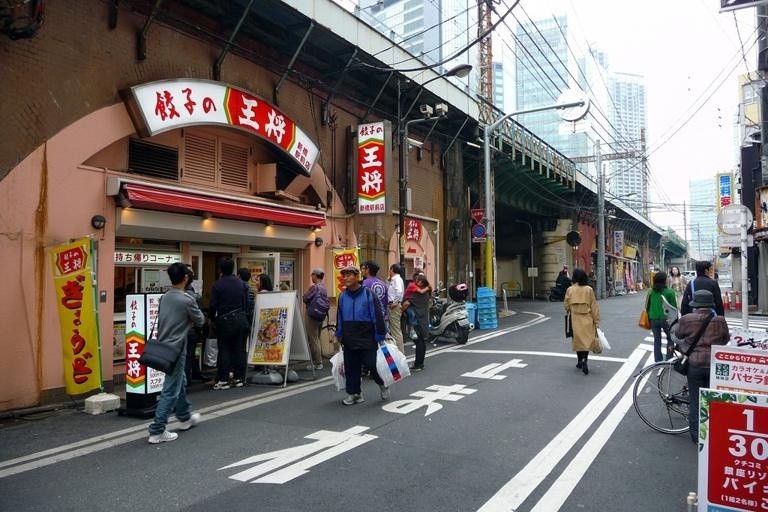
[734,290,741,312]
[723,292,731,313]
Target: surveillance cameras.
[420,104,434,118]
[436,103,449,116]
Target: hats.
[311,268,325,276]
[339,265,360,275]
[688,289,717,308]
[564,265,569,269]
[413,270,424,281]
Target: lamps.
[315,237,323,247]
[116,192,132,210]
[92,215,106,230]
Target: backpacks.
[307,283,331,323]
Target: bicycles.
[632,359,694,435]
[321,324,340,359]
[656,333,768,421]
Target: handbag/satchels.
[376,337,411,387]
[210,282,248,335]
[673,350,690,376]
[136,339,182,376]
[592,327,604,354]
[329,342,363,391]
[564,309,574,339]
[638,288,652,330]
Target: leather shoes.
[576,359,590,375]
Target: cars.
[679,270,697,281]
[718,270,731,284]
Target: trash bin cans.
[466,303,477,330]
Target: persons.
[562,266,600,374]
[680,260,725,317]
[208,260,273,390]
[148,262,206,442]
[668,265,689,312]
[335,267,390,404]
[302,268,327,369]
[359,261,431,371]
[669,289,730,444]
[556,264,571,287]
[645,271,678,361]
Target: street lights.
[391,60,476,288]
[515,219,536,302]
[598,192,637,300]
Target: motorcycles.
[429,281,473,346]
[548,278,574,303]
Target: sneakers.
[212,380,230,392]
[380,385,392,400]
[409,363,425,371]
[148,430,179,444]
[232,379,243,388]
[177,412,203,431]
[342,393,365,406]
